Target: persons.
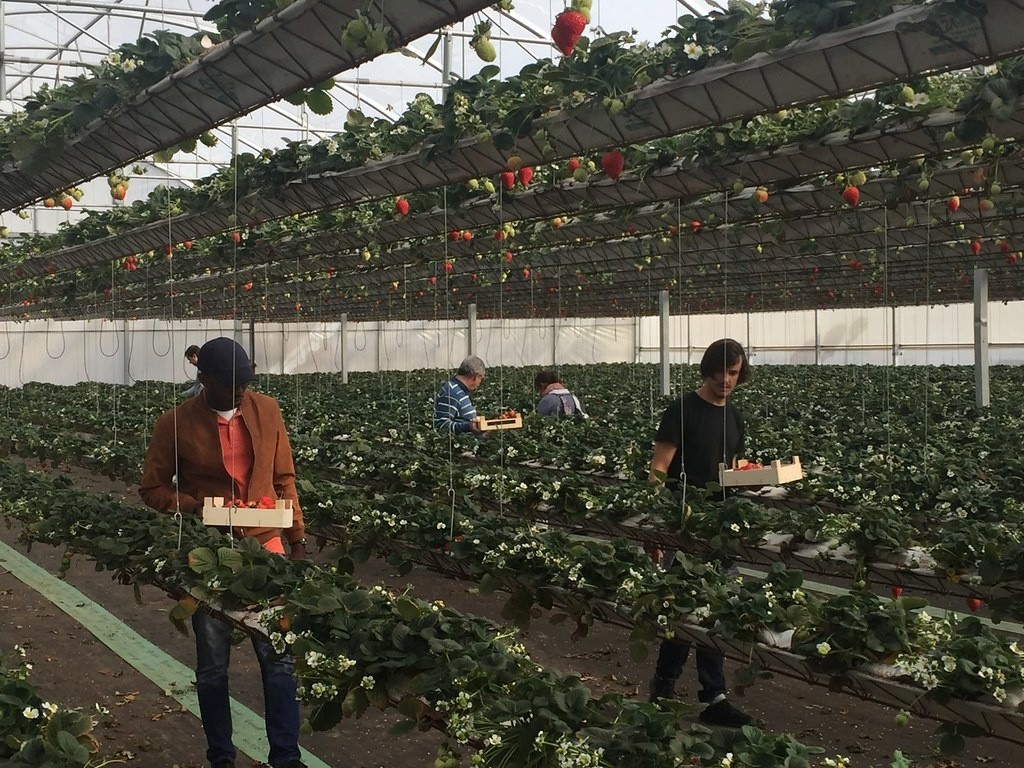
[138,337,308,768]
[535,370,581,417]
[434,355,485,434]
[649,339,757,728]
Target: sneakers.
[273,755,308,767]
[696,694,754,730]
[650,692,676,715]
[207,752,236,767]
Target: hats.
[199,338,259,388]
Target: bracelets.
[290,537,308,546]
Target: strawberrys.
[891,586,903,598]
[732,462,763,471]
[221,497,277,510]
[967,598,981,613]
[494,409,516,423]
[0,0,1024,308]
